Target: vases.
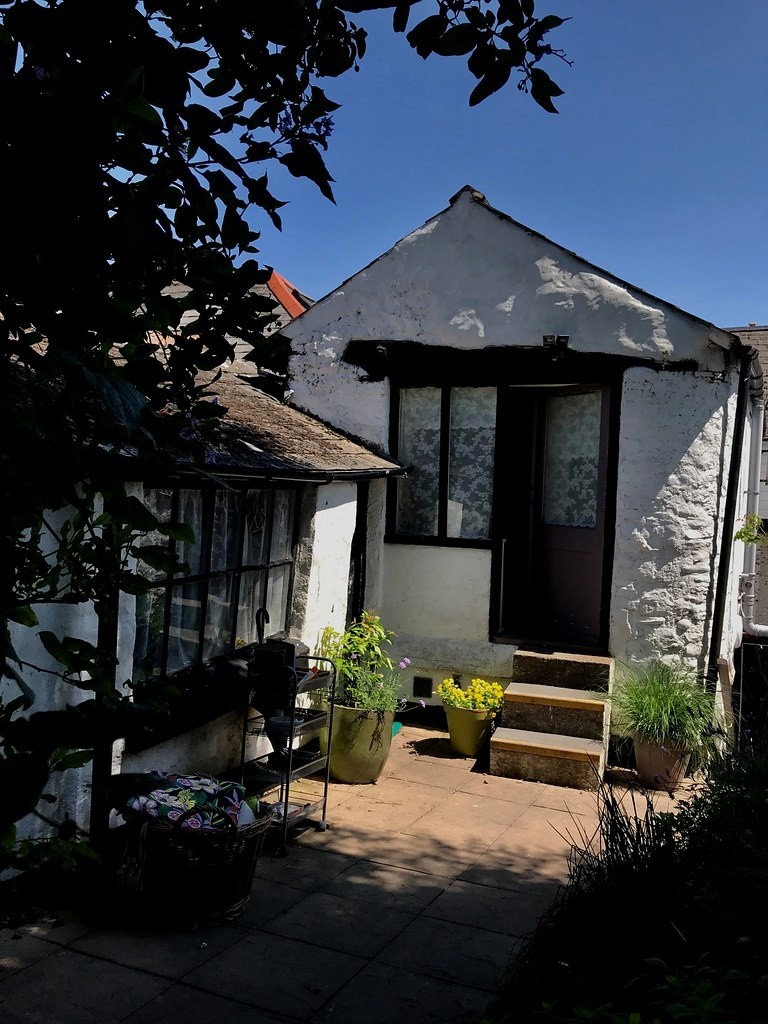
[319,695,395,784]
[443,700,493,755]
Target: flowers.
[322,606,426,715]
[432,679,505,718]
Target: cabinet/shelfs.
[239,655,336,857]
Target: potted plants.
[587,650,747,794]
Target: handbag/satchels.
[116,771,246,829]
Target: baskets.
[122,770,275,927]
